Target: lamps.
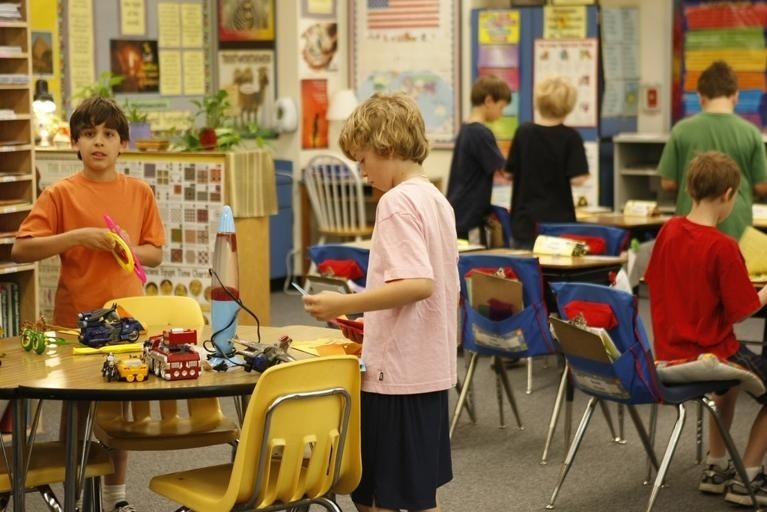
[30,80,56,148]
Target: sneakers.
[724,470,767,507]
[698,457,737,496]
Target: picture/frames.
[218,48,276,140]
[302,0,337,21]
[216,0,275,47]
[348,0,462,150]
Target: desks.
[0,324,367,512]
[34,140,272,331]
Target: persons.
[33,79,57,138]
[11,99,166,512]
[645,151,767,505]
[659,62,767,244]
[302,92,460,511]
[447,76,513,241]
[504,80,590,250]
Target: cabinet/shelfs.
[0,1,38,439]
[610,132,767,212]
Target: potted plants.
[122,97,152,149]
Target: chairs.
[0,435,119,512]
[148,357,364,512]
[90,296,241,466]
